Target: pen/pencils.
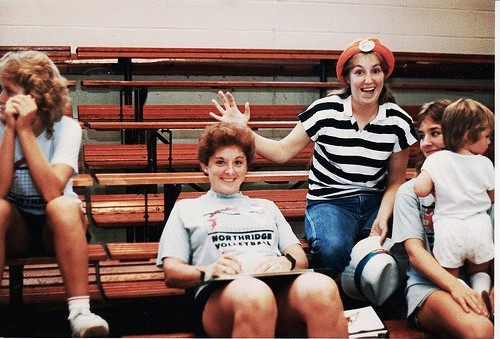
[216,245,244,275]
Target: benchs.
[0,46,495,339]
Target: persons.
[209,37,419,276]
[0,51,109,337]
[157,122,349,339]
[413,99,495,317]
[389,99,495,339]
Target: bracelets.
[283,254,296,271]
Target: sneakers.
[68,311,108,338]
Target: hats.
[336,38,394,85]
[340,237,408,305]
